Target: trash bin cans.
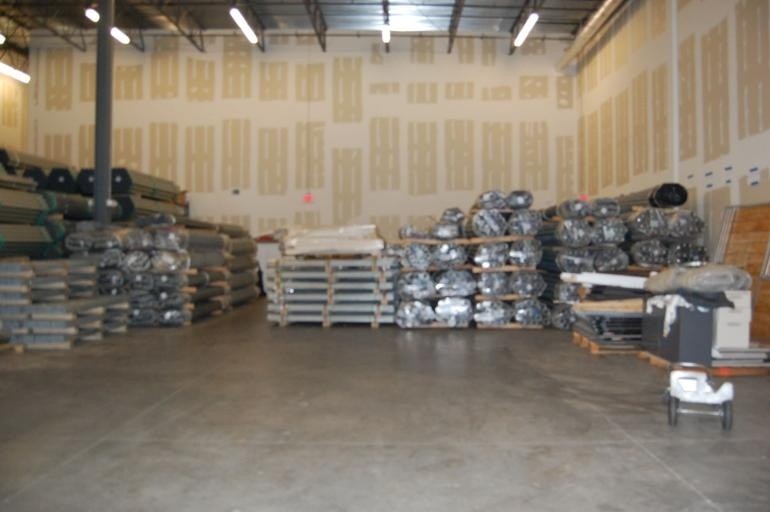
[641,288,715,368]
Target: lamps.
[513,12,539,48]
[382,24,390,43]
[0,62,31,84]
[0,33,6,45]
[229,8,258,44]
[84,8,131,45]
